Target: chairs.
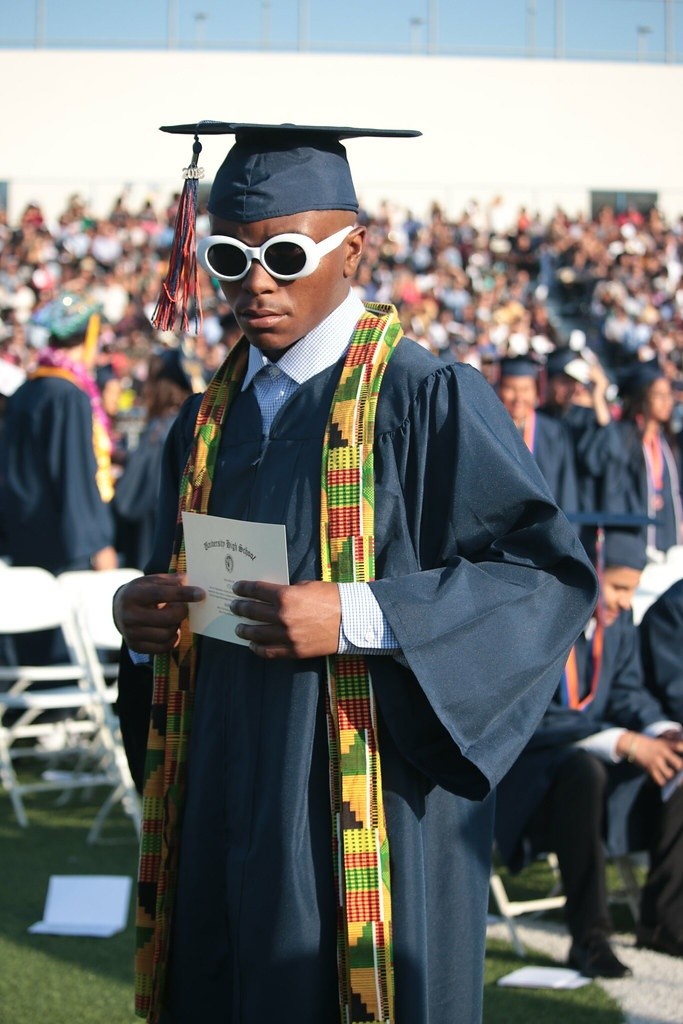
[476,858,639,957]
[0,566,164,844]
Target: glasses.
[196,223,353,281]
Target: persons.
[111,119,597,1024]
[497,516,683,979]
[1,186,683,769]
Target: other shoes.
[637,931,683,957]
[566,943,632,978]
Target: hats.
[151,121,423,334]
[29,289,104,368]
[161,345,200,392]
[571,515,662,569]
[615,356,662,400]
[483,354,545,376]
